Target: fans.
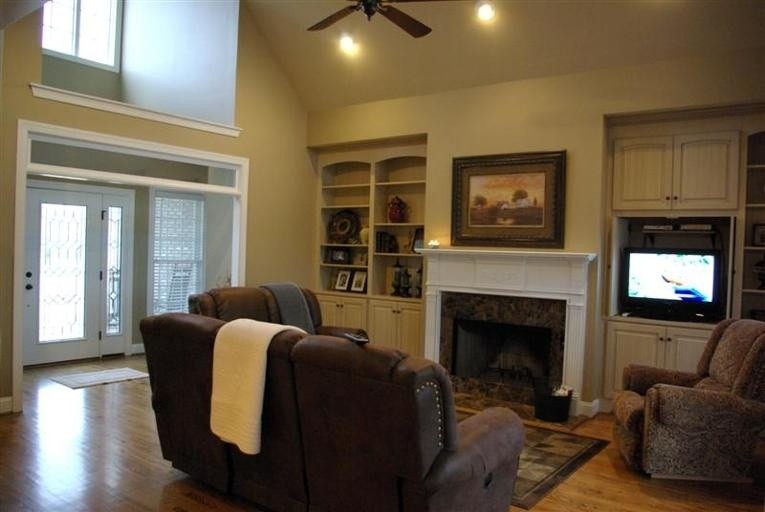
[306,0,469,37]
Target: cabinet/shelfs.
[605,121,749,215]
[308,142,426,303]
[315,293,368,333]
[367,298,425,357]
[602,318,715,401]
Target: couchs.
[139,313,524,512]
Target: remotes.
[343,332,372,349]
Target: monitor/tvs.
[619,248,727,324]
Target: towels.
[208,319,309,455]
[258,283,314,334]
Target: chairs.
[187,285,369,344]
[613,318,765,483]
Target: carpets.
[49,366,150,389]
[453,408,612,510]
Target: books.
[376,231,398,253]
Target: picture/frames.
[351,270,367,292]
[751,223,765,247]
[334,270,351,292]
[450,148,566,249]
